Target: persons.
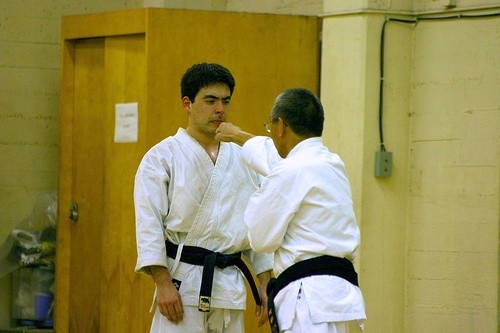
[215,88,367,333]
[133,62,274,333]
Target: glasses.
[264,117,287,133]
